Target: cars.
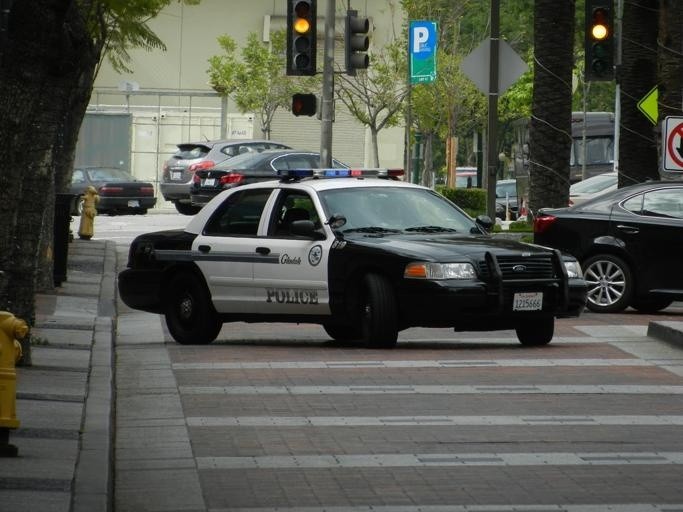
[455,163,683,316]
[190,147,353,208]
[114,165,593,351]
[69,165,157,218]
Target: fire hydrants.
[77,185,101,241]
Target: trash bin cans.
[53,193,80,287]
[70,193,84,216]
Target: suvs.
[157,135,295,216]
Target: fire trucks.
[0,309,31,456]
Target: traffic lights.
[586,0,616,82]
[286,1,318,78]
[343,8,370,77]
[292,92,318,116]
